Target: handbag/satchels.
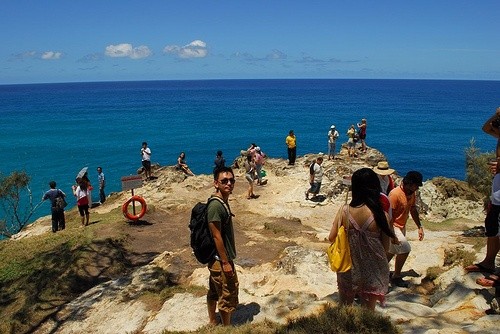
[327,207,352,273]
[89,185,93,190]
[56,189,67,208]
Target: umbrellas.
[76,166,89,178]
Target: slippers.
[465,263,495,272]
[477,275,500,286]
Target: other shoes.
[305,192,309,200]
[215,313,223,323]
[79,225,85,228]
[251,195,259,198]
[391,277,408,287]
[247,197,251,199]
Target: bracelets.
[418,227,423,229]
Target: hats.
[255,147,260,151]
[373,161,394,175]
[331,125,335,128]
[403,171,423,186]
[241,150,247,155]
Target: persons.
[286,130,297,165]
[464,106,500,286]
[385,171,424,287]
[42,181,66,233]
[305,157,323,200]
[140,142,151,180]
[177,152,194,176]
[207,166,239,326]
[328,125,339,160]
[373,161,395,195]
[244,143,265,199]
[214,150,226,166]
[357,119,367,153]
[328,167,398,313]
[346,125,357,157]
[71,172,92,226]
[97,167,106,204]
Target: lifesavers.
[122,195,147,220]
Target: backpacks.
[189,196,235,265]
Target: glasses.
[220,178,235,184]
